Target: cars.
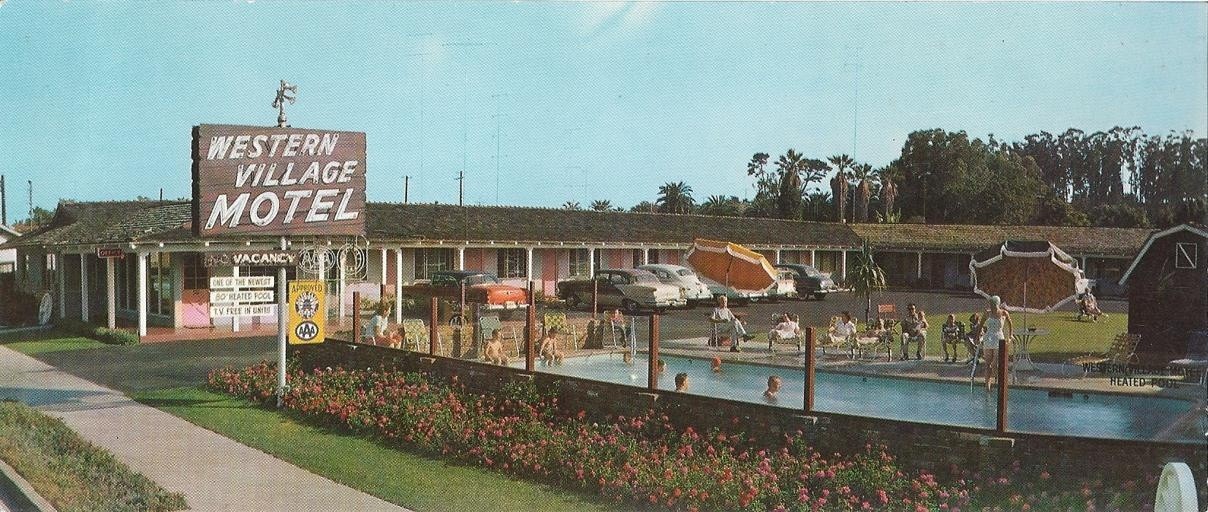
[555,263,834,313]
[402,270,530,319]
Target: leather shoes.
[742,333,755,343]
[729,345,742,353]
[900,353,909,361]
[916,350,924,360]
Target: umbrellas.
[684,234,777,311]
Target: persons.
[941,314,960,361]
[900,302,929,361]
[484,327,510,366]
[366,299,400,348]
[770,312,800,338]
[609,308,631,346]
[710,294,756,352]
[675,371,689,391]
[710,355,722,370]
[909,311,924,334]
[658,358,666,371]
[1081,287,1099,324]
[853,320,889,344]
[978,295,1015,390]
[765,375,781,396]
[824,310,858,344]
[538,326,564,366]
[623,352,634,364]
[964,314,984,363]
